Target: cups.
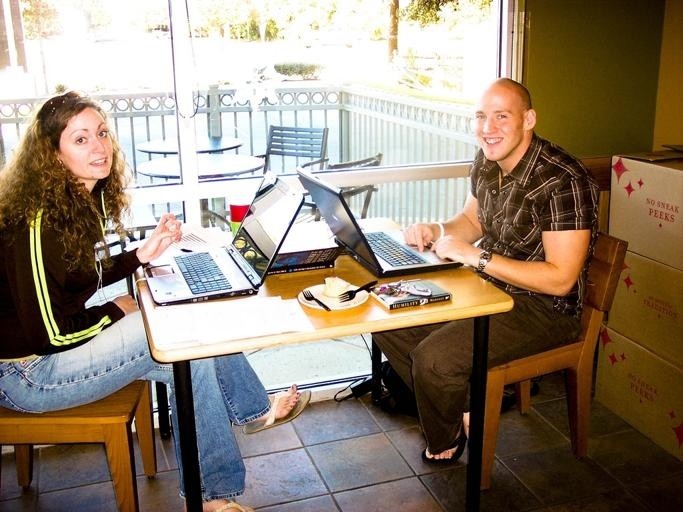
[228,203,249,237]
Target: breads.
[323,277,347,296]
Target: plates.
[298,283,369,310]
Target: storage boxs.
[590,141,683,466]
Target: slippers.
[213,496,256,512]
[421,422,469,465]
[242,389,312,435]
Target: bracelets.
[435,223,444,239]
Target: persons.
[0,92,311,512]
[374,77,600,466]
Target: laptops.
[296,169,464,278]
[142,170,304,308]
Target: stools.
[92,238,135,299]
[102,207,165,243]
[0,373,160,512]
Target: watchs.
[477,249,491,272]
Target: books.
[267,246,348,277]
[370,280,452,310]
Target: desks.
[133,155,268,229]
[135,136,246,158]
[122,212,515,512]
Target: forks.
[338,280,378,305]
[301,288,332,314]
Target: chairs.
[478,232,630,493]
[290,151,387,225]
[260,123,330,215]
